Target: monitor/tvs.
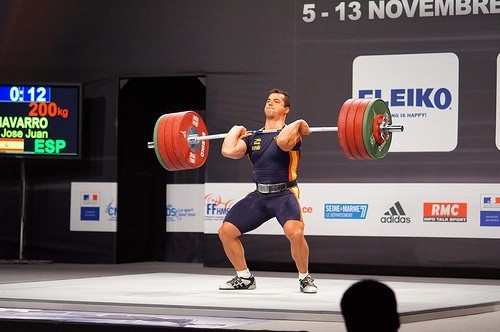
[0,82,82,160]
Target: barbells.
[148,98,404,171]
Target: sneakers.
[299,275,318,293]
[219,273,256,290]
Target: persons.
[218,89,319,294]
[340,279,402,332]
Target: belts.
[256,180,297,194]
